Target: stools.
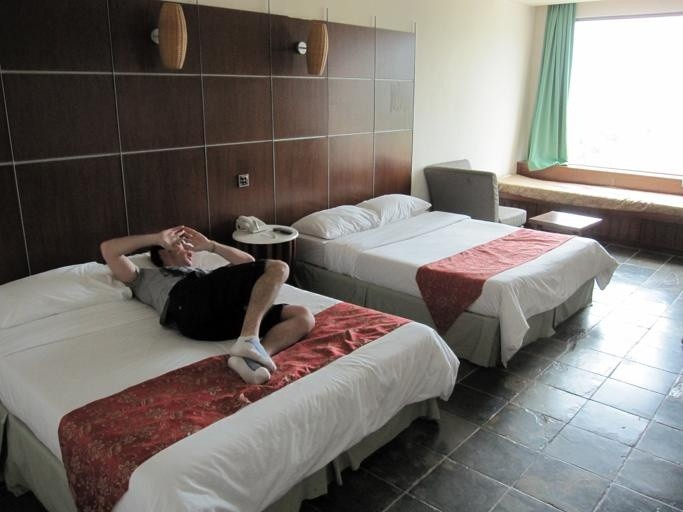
[529,210,604,239]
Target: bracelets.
[207,240,215,252]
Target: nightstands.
[232,225,301,288]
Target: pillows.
[0,260,132,330]
[126,243,232,276]
[290,205,380,239]
[356,194,432,225]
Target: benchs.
[497,158,683,219]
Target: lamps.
[297,23,329,76]
[151,3,188,70]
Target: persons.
[100,224,316,385]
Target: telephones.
[235,214,269,234]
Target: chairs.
[424,158,527,228]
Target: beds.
[0,282,460,512]
[291,209,618,368]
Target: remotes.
[272,225,292,235]
